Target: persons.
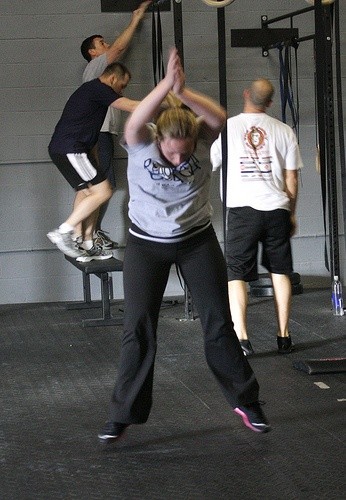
[98,48,273,442]
[71,0,151,251]
[210,77,303,358]
[48,62,142,263]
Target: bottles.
[331,276,344,317]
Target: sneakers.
[233,399,272,433]
[98,421,129,440]
[46,223,119,263]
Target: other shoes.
[240,339,254,358]
[277,334,296,353]
